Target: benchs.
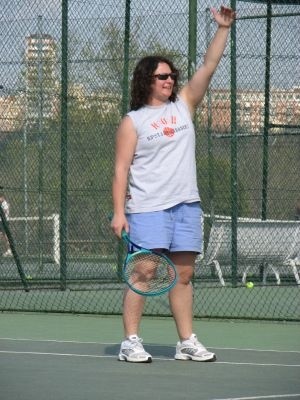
[191,216,300,291]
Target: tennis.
[246,281,254,288]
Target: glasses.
[152,72,179,80]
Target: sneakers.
[118,335,152,363]
[175,334,216,362]
[1,250,14,258]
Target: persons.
[110,5,236,364]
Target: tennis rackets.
[107,209,177,297]
